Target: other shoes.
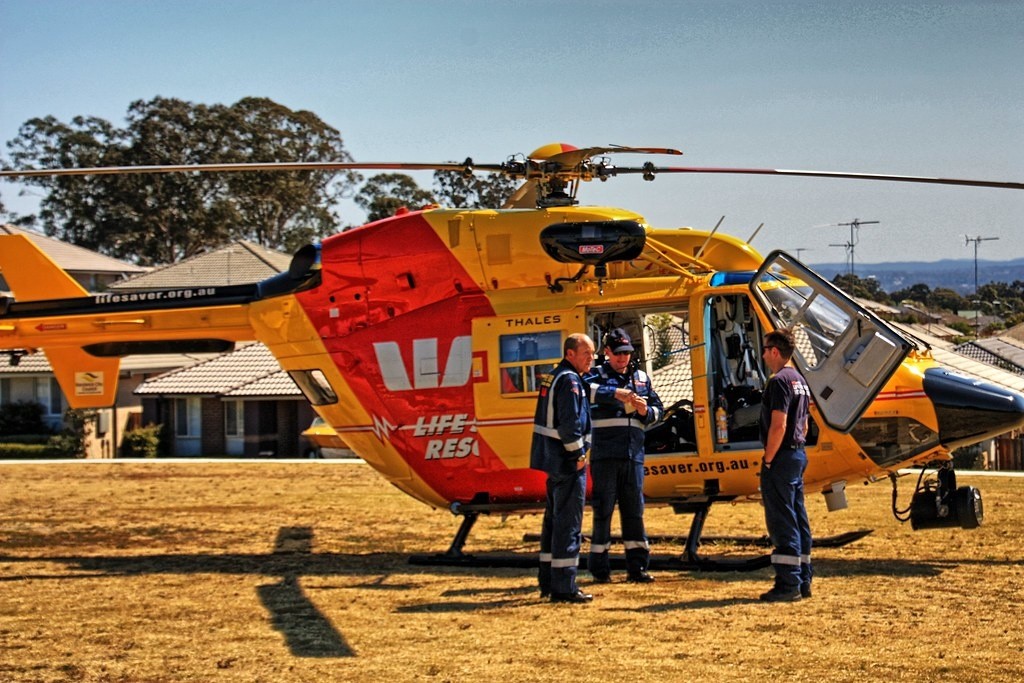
[594,576,610,583]
[760,585,811,602]
[627,572,654,582]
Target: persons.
[529,332,594,605]
[580,329,663,583]
[758,329,812,603]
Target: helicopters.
[0,141,1024,571]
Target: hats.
[604,328,634,353]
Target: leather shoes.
[541,589,593,603]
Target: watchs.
[574,455,587,461]
[761,455,772,466]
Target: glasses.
[761,346,781,354]
[608,349,630,355]
